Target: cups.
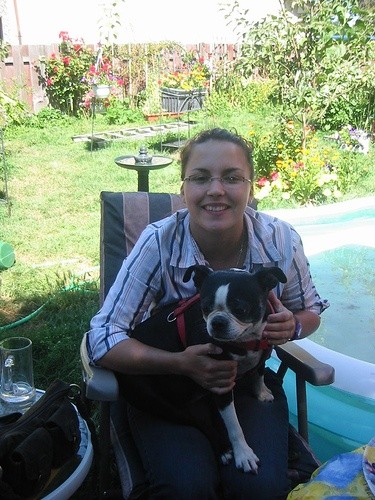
[0,336,37,403]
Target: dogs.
[118,266,287,474]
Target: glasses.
[184,173,254,186]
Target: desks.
[0,387,94,500]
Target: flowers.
[163,59,211,89]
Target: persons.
[86,127,330,500]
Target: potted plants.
[141,74,178,122]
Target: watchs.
[288,316,302,341]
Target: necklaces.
[236,219,246,268]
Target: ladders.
[71,119,200,148]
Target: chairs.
[79,190,335,500]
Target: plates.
[362,436,375,497]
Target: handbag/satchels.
[0,380,83,500]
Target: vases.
[159,86,205,111]
